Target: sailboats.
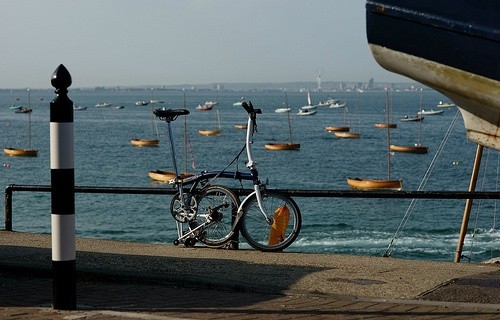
[323,93,351,132]
[4,88,37,157]
[334,92,361,138]
[390,87,428,153]
[347,85,403,188]
[301,90,318,108]
[197,97,221,135]
[264,91,301,150]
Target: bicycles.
[152,99,301,252]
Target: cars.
[9,100,163,113]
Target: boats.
[400,115,425,122]
[234,124,247,129]
[131,139,160,147]
[275,107,291,113]
[418,109,444,115]
[148,170,194,183]
[437,101,455,107]
[233,102,243,106]
[375,124,398,128]
[296,96,346,116]
[365,0,500,149]
